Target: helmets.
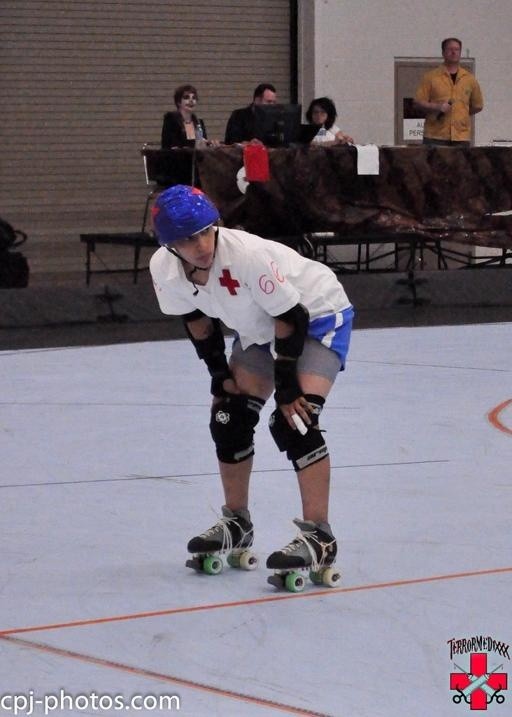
[153,185,218,246]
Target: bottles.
[316,125,327,147]
[194,123,204,149]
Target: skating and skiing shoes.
[188,506,257,575]
[266,519,341,593]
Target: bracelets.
[273,357,300,390]
[205,355,228,374]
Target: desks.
[77,139,509,305]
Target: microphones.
[196,119,202,139]
[437,98,454,120]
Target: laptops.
[282,124,321,147]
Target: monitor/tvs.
[254,103,301,145]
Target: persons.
[150,181,353,594]
[158,84,218,149]
[224,82,280,147]
[305,95,353,145]
[412,37,486,146]
